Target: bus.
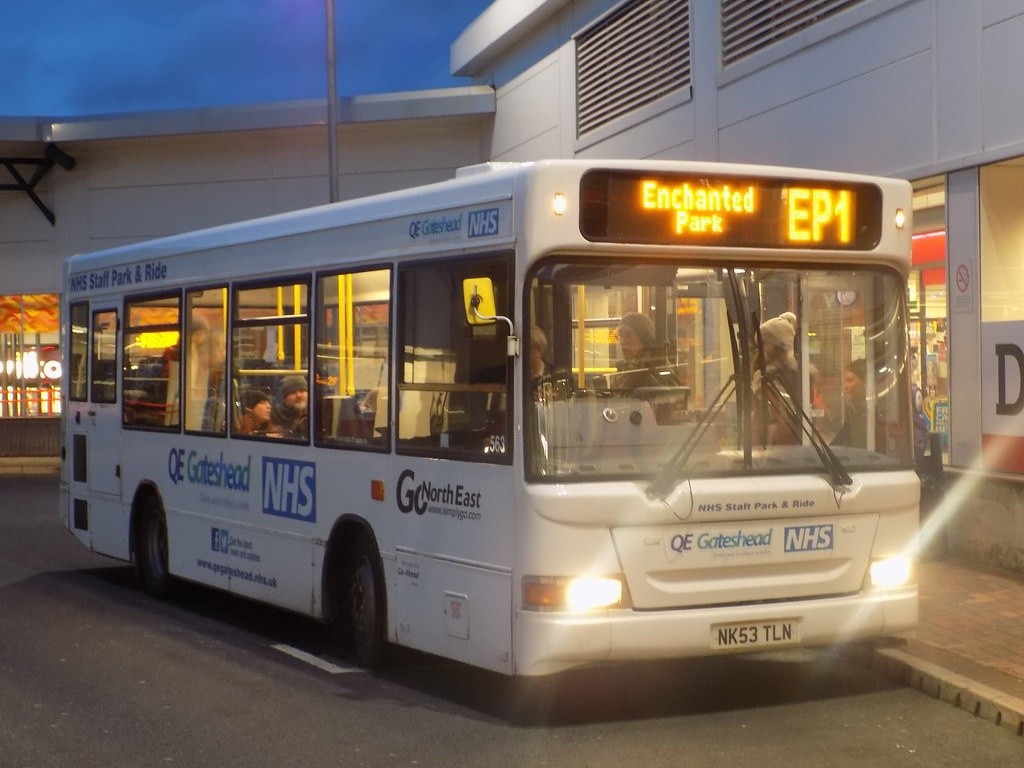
[56,147,931,684]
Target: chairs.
[68,352,272,436]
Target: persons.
[750,312,798,445]
[830,359,886,452]
[163,320,225,429]
[265,376,308,438]
[360,388,380,434]
[219,390,271,437]
[613,314,681,422]
[482,324,559,451]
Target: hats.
[847,360,867,382]
[754,311,797,352]
[622,311,656,339]
[280,375,308,396]
[239,390,272,407]
